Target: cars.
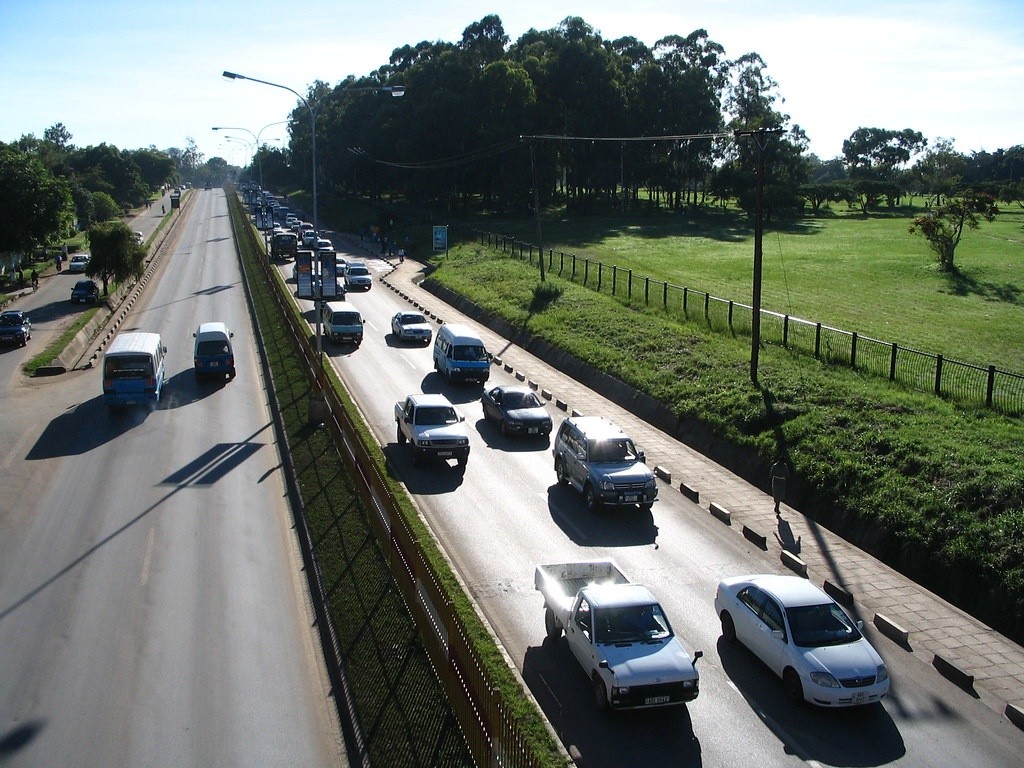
[70,281,99,304]
[714,574,890,710]
[392,311,433,343]
[238,179,372,299]
[133,231,145,245]
[69,255,91,272]
[175,182,191,194]
[482,384,553,440]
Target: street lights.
[223,70,405,352]
[212,119,301,267]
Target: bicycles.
[33,280,38,294]
[57,262,61,274]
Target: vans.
[433,324,490,388]
[192,322,237,381]
[322,302,366,347]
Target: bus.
[103,332,167,406]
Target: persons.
[162,205,165,214]
[925,201,928,207]
[301,212,305,221]
[284,194,287,201]
[611,198,623,209]
[382,237,404,263]
[864,205,866,212]
[56,254,62,270]
[680,204,689,215]
[328,224,333,231]
[349,217,394,242]
[10,268,39,292]
[927,210,932,218]
[168,185,169,192]
[75,257,79,261]
[579,606,652,632]
[62,242,67,261]
[427,209,433,224]
[146,200,151,209]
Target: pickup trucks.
[534,561,703,712]
[395,394,470,469]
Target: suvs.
[552,416,659,513]
[0,310,31,346]
